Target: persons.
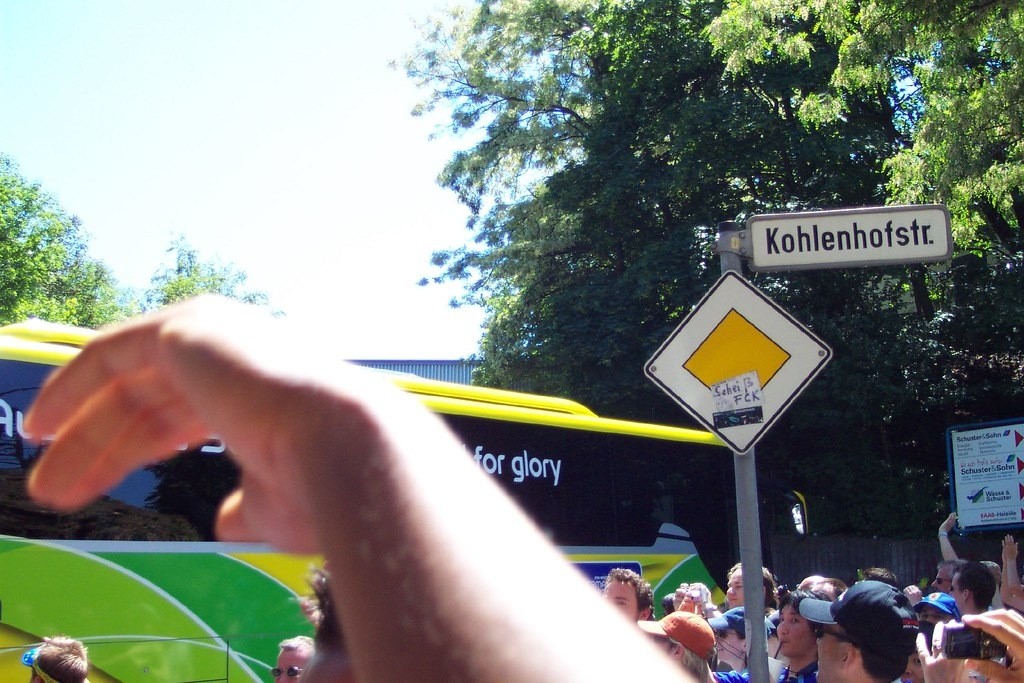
[22,634,90,683]
[271,636,316,683]
[22,294,700,683]
[603,512,1024,683]
[799,580,919,683]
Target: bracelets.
[938,531,948,537]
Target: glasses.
[934,577,952,584]
[711,628,738,639]
[270,666,302,679]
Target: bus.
[1,323,814,683]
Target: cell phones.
[942,625,1007,660]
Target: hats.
[709,606,776,641]
[21,644,60,683]
[637,611,715,659]
[799,579,920,657]
[766,609,782,636]
[913,592,961,622]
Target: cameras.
[690,586,706,606]
